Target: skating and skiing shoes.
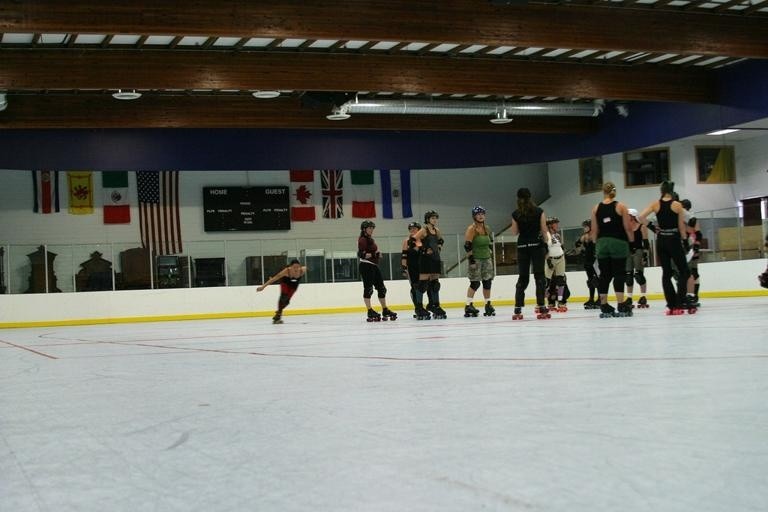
[425,303,448,319]
[413,305,431,320]
[463,302,480,317]
[583,298,601,309]
[271,314,284,324]
[547,304,568,313]
[366,308,381,322]
[382,308,398,321]
[483,301,496,316]
[664,293,701,316]
[511,307,523,320]
[615,296,635,317]
[599,303,616,318]
[534,304,551,319]
[637,295,650,308]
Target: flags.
[320,169,342,218]
[32,169,59,214]
[379,167,412,219]
[64,171,93,216]
[289,169,315,221]
[136,169,181,256]
[101,169,131,225]
[349,168,375,218]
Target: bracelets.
[467,255,476,265]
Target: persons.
[462,205,496,317]
[543,216,567,312]
[408,210,447,320]
[509,188,551,320]
[561,180,704,317]
[356,220,397,322]
[400,222,431,318]
[256,259,309,324]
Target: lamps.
[111,89,143,102]
[490,108,514,126]
[324,105,352,122]
[249,90,281,100]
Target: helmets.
[407,222,421,230]
[360,220,376,230]
[472,206,486,217]
[424,209,439,218]
[582,219,591,233]
[546,216,560,225]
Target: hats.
[680,197,691,211]
[627,209,641,224]
